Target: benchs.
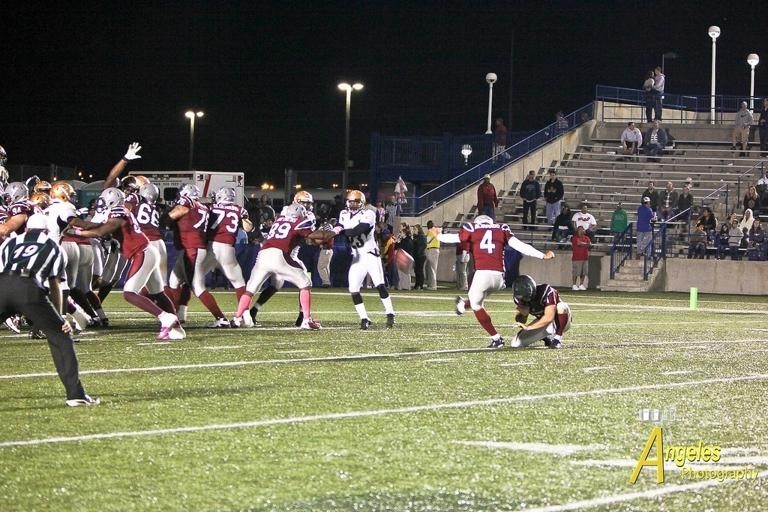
[444,137,768,253]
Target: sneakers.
[4,316,22,333]
[543,336,562,349]
[155,312,186,340]
[425,286,437,291]
[386,313,395,330]
[453,285,468,291]
[72,315,109,332]
[65,394,101,407]
[572,285,580,290]
[28,327,45,339]
[578,285,586,291]
[487,336,505,348]
[455,295,466,316]
[294,317,322,331]
[360,319,372,330]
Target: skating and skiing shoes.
[205,313,261,328]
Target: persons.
[492,118,513,169]
[653,66,665,121]
[730,101,754,157]
[555,111,568,135]
[620,122,643,155]
[642,121,667,163]
[642,71,655,122]
[758,97,768,157]
[0,142,768,348]
[1,213,97,407]
[581,111,590,123]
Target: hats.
[26,212,52,233]
[641,197,651,202]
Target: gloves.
[331,226,343,236]
[59,314,74,336]
[121,142,142,163]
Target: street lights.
[460,144,474,168]
[184,108,205,169]
[337,81,364,203]
[708,25,722,126]
[747,53,761,117]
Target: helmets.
[96,174,160,214]
[5,175,78,211]
[512,275,537,307]
[346,190,366,214]
[175,183,200,201]
[215,187,235,204]
[473,215,494,226]
[289,191,314,219]
